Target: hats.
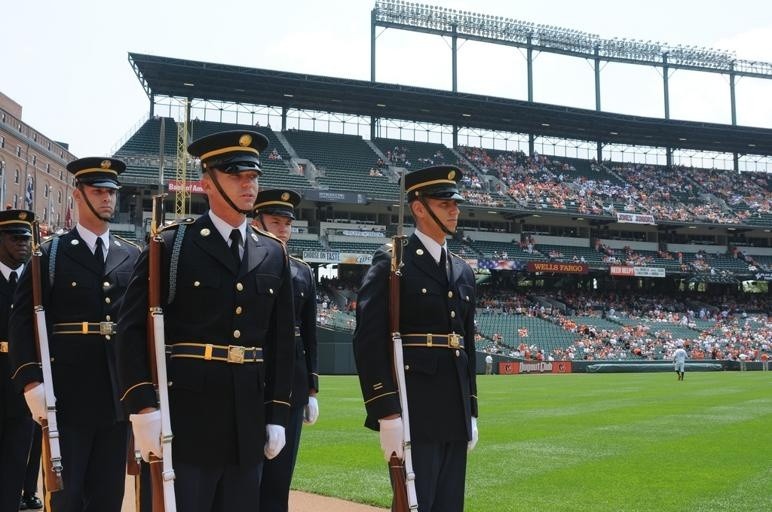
[66,157,126,190]
[0,210,34,238]
[398,165,467,203]
[251,188,301,220]
[188,130,269,175]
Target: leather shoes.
[18,493,43,509]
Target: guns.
[149,195,177,512]
[389,236,416,511]
[29,221,63,493]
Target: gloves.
[467,417,478,450]
[264,423,288,460]
[22,383,57,425]
[302,396,320,425]
[380,417,405,463]
[129,411,165,463]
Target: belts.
[170,341,264,365]
[401,331,467,350]
[52,321,118,336]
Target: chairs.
[109,115,772,364]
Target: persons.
[739,353,748,371]
[252,188,319,512]
[8,157,143,512]
[19,423,42,510]
[315,274,357,330]
[761,352,769,371]
[369,143,772,361]
[671,345,689,380]
[353,164,479,512]
[485,353,493,375]
[114,129,295,512]
[0,209,35,512]
[268,148,283,161]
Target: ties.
[230,228,241,268]
[438,248,448,281]
[94,237,105,273]
[9,272,18,293]
